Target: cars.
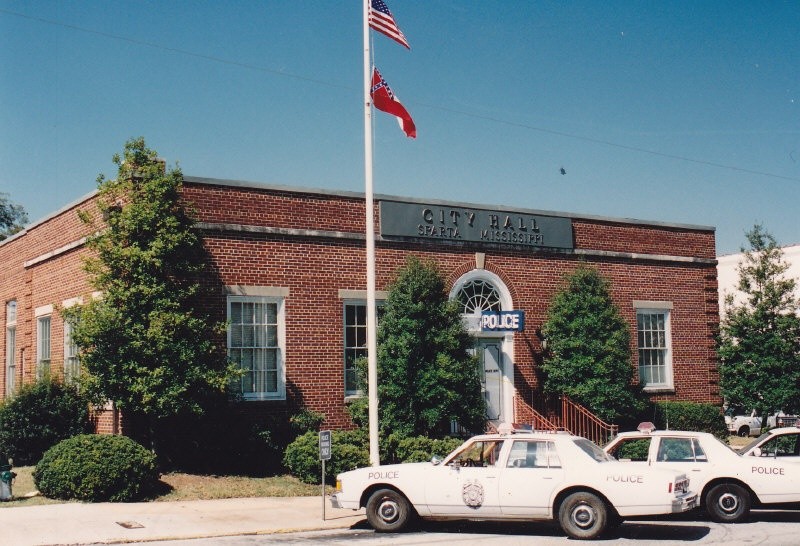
[724,404,800,437]
[604,422,800,522]
[330,423,698,541]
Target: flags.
[367,0,411,50]
[370,67,417,139]
